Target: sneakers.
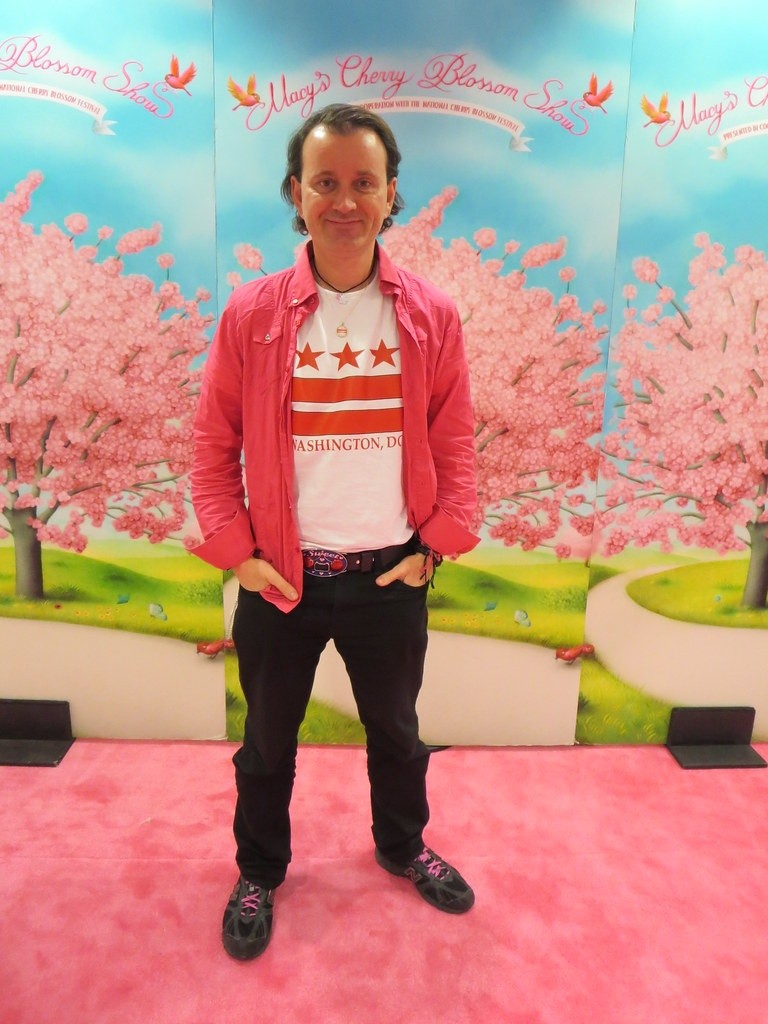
[375,839,476,914]
[222,873,273,960]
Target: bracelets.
[417,544,442,566]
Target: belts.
[302,541,411,578]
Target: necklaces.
[314,269,373,338]
[313,258,374,304]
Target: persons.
[192,103,480,959]
